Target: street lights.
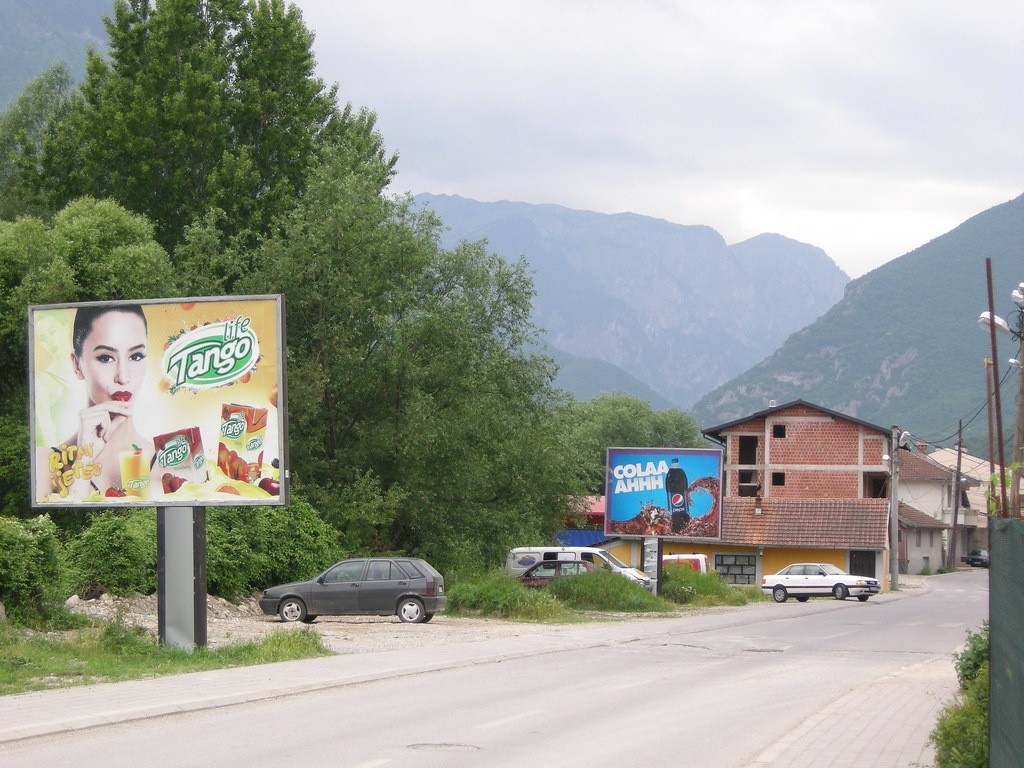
[978,281,1024,516]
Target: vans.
[503,546,654,595]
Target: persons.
[43,304,155,498]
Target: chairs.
[368,568,383,581]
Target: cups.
[120,447,150,496]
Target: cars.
[515,559,601,589]
[760,562,881,602]
[966,548,990,568]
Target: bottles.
[666,457,691,534]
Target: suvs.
[257,556,445,623]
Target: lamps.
[753,495,764,515]
